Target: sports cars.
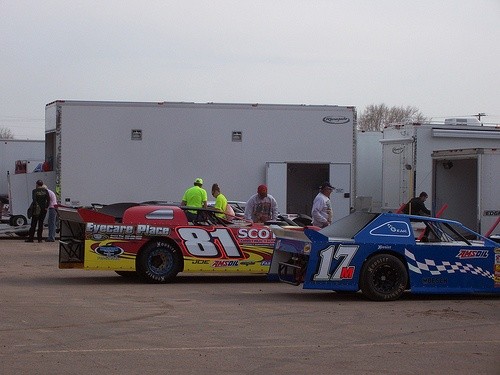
[266,208,500,302]
[55,201,323,284]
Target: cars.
[208,202,248,218]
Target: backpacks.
[226,204,235,221]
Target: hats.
[258,185,267,197]
[321,183,335,190]
[195,178,203,184]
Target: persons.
[244,184,278,223]
[24,179,50,242]
[212,184,227,220]
[180,177,207,222]
[41,184,58,242]
[399,191,431,216]
[311,181,333,228]
